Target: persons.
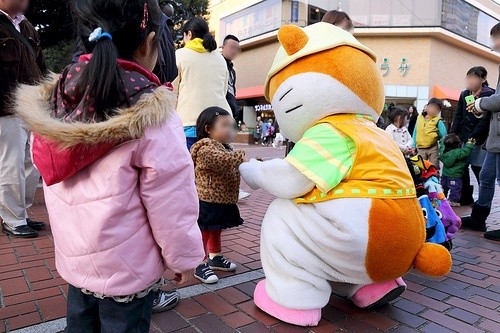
[221,34,240,125]
[384,103,414,159]
[454,66,496,205]
[441,101,453,133]
[190,106,263,284]
[461,22,500,241]
[0,0,49,238]
[437,133,476,207]
[171,17,233,152]
[254,115,277,146]
[407,104,419,136]
[412,97,447,176]
[376,102,396,130]
[14,0,207,333]
[73,0,181,314]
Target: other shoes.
[448,200,460,207]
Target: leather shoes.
[2,223,38,238]
[26,218,46,230]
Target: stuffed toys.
[240,10,452,327]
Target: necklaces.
[470,89,481,98]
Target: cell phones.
[465,95,476,112]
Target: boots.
[458,185,474,204]
[484,229,500,240]
[460,202,490,232]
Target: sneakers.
[194,264,219,284]
[238,189,251,199]
[152,289,180,314]
[208,256,237,271]
